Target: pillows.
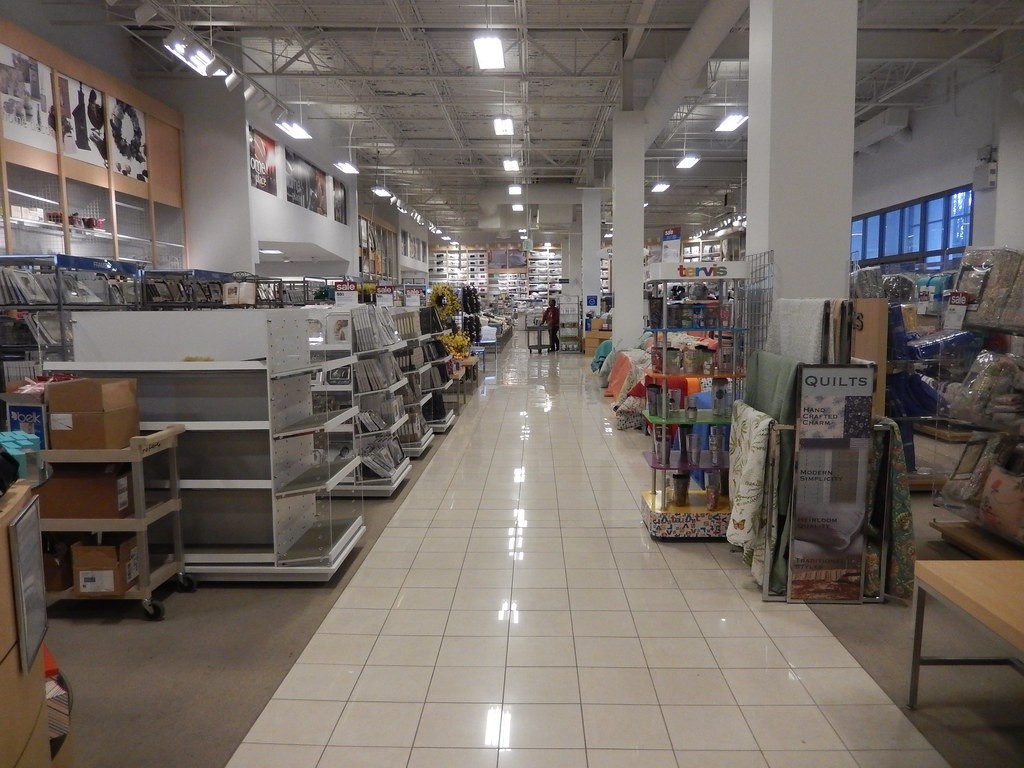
[794,501,865,550]
[976,463,1024,545]
[791,540,854,571]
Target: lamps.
[105,0,259,101]
[332,121,360,174]
[370,170,460,246]
[473,0,532,242]
[674,128,700,169]
[603,224,613,238]
[271,79,312,140]
[650,160,670,192]
[714,62,748,132]
[257,93,270,112]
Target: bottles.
[660,470,724,509]
[649,343,733,376]
[646,288,734,329]
[652,424,726,467]
[647,377,738,420]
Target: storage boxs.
[45,677,71,740]
[32,468,132,519]
[560,329,578,338]
[585,347,597,356]
[585,331,611,339]
[584,339,599,348]
[0,377,83,449]
[72,534,139,597]
[559,304,579,314]
[591,319,607,329]
[557,296,579,303]
[48,377,139,450]
[559,314,578,323]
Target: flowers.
[437,332,470,371]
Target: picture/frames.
[388,436,407,464]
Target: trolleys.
[41,424,198,621]
[525,312,551,354]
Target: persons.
[600,308,610,319]
[542,300,559,352]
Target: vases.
[648,297,663,329]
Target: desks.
[449,366,466,415]
[908,560,1024,709]
[462,356,479,396]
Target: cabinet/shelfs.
[0,258,1024,622]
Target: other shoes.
[550,348,555,352]
[556,347,559,351]
